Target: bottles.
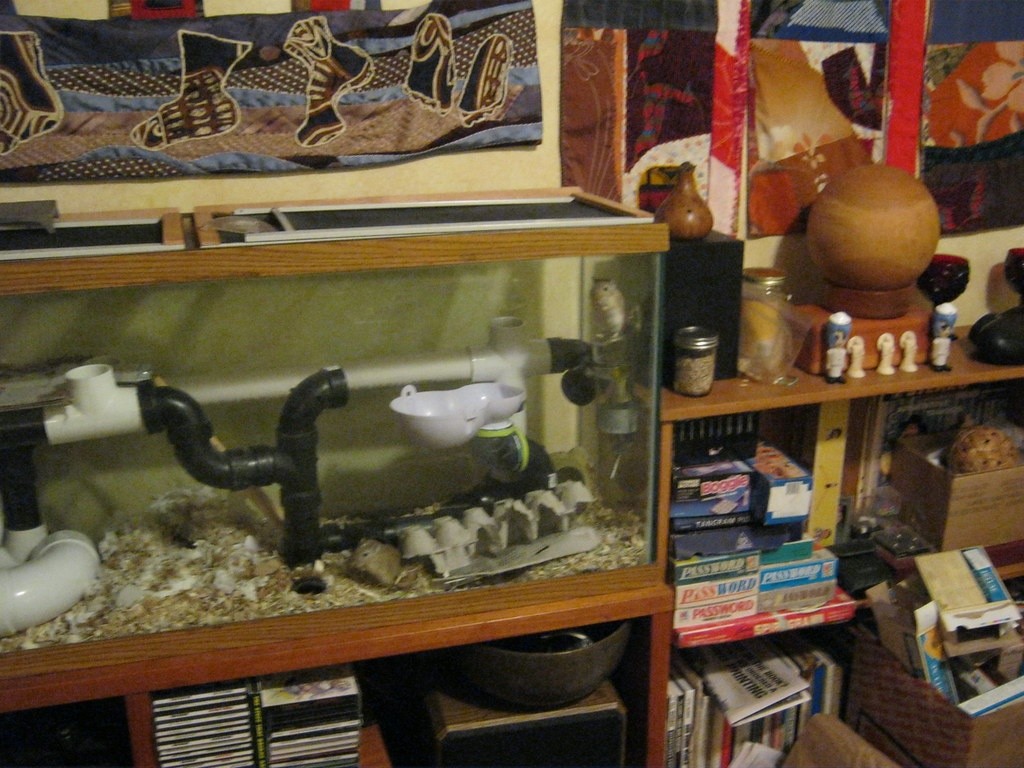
[740,267,790,383]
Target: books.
[664,635,843,768]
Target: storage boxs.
[842,622,1024,768]
[887,425,1023,552]
[670,445,838,631]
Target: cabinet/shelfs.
[657,322,1024,768]
